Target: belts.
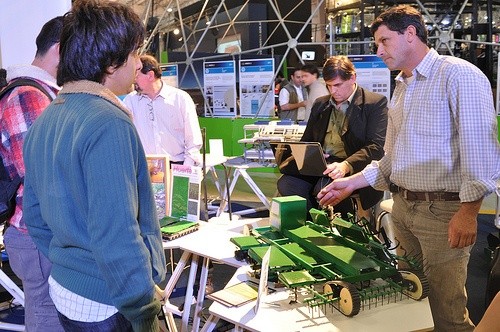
[169,161,184,169]
[398,187,460,201]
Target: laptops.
[269,140,333,178]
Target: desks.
[215,154,279,217]
[200,153,237,212]
[161,220,211,332]
[179,217,436,332]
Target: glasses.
[147,102,153,120]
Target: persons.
[316,4,500,332]
[300,64,330,121]
[20,0,166,332]
[0,16,65,332]
[275,70,289,116]
[276,54,388,222]
[279,68,309,122]
[123,55,203,168]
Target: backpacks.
[0,77,54,224]
